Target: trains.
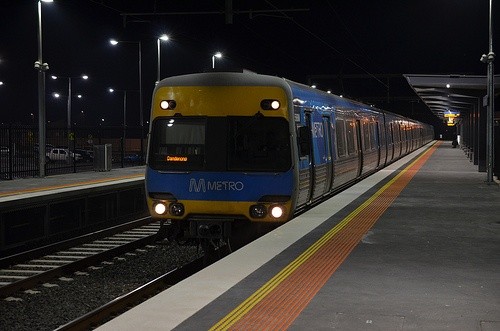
[143,68,435,265]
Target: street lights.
[212,51,222,69]
[109,38,146,165]
[108,87,139,140]
[36,0,55,177]
[50,73,89,150]
[155,33,169,83]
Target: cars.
[47,147,83,162]
